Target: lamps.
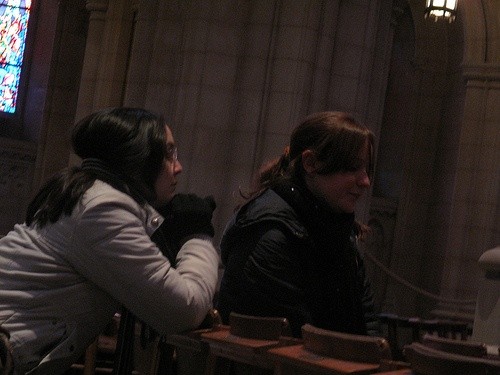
[424,0,460,24]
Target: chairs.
[71,308,500,375]
[0,324,25,375]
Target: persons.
[217,112,378,337]
[0,107,225,375]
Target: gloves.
[165,191,217,240]
